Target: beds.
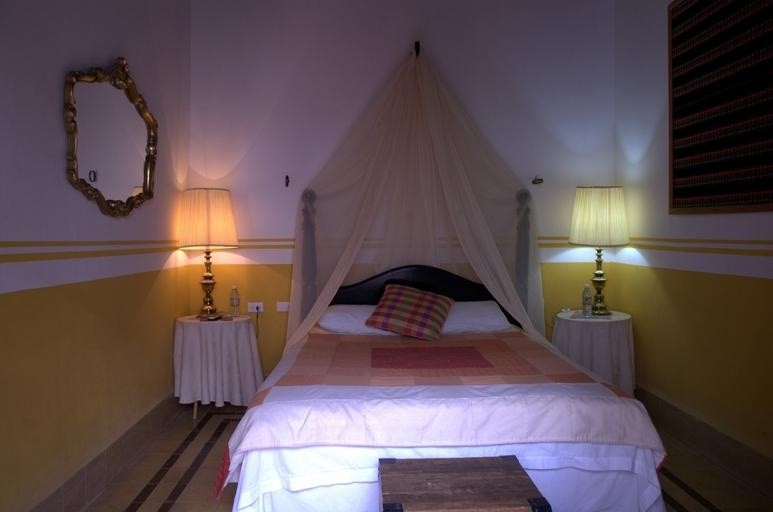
[212,188,669,511]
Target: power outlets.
[247,302,263,313]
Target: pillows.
[440,300,511,335]
[364,284,456,343]
[315,303,400,337]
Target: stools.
[375,453,554,512]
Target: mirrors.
[62,57,158,217]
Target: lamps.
[175,188,240,319]
[568,186,630,315]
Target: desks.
[171,315,264,421]
[550,308,638,395]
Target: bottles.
[582,284,593,318]
[230,284,241,318]
